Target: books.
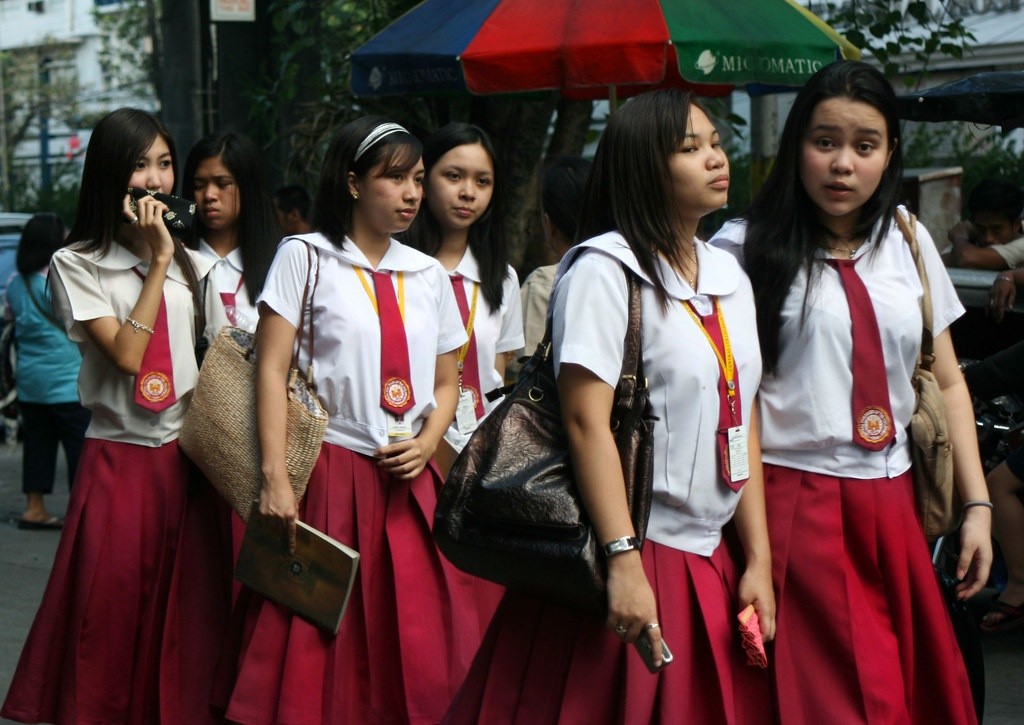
[234,498,360,635]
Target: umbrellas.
[891,68,1024,128]
[344,0,868,114]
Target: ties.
[219,274,248,330]
[446,272,486,434]
[135,264,177,415]
[366,268,415,417]
[827,260,894,453]
[688,298,750,495]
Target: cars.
[0,212,36,302]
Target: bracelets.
[995,276,1015,284]
[126,316,154,336]
[961,500,993,510]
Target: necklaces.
[810,236,866,256]
[670,243,697,291]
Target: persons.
[418,121,525,644]
[432,89,783,725]
[940,183,1024,270]
[0,109,211,725]
[708,58,994,725]
[963,268,1024,636]
[267,183,316,238]
[213,112,478,725]
[176,134,272,610]
[520,155,593,367]
[6,213,93,531]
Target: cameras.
[635,631,673,674]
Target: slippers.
[979,589,1024,635]
[19,514,61,530]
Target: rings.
[130,217,139,226]
[615,625,628,634]
[645,623,660,630]
[990,300,994,305]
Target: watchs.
[601,535,641,557]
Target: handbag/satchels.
[431,247,660,599]
[178,239,329,526]
[896,204,961,536]
